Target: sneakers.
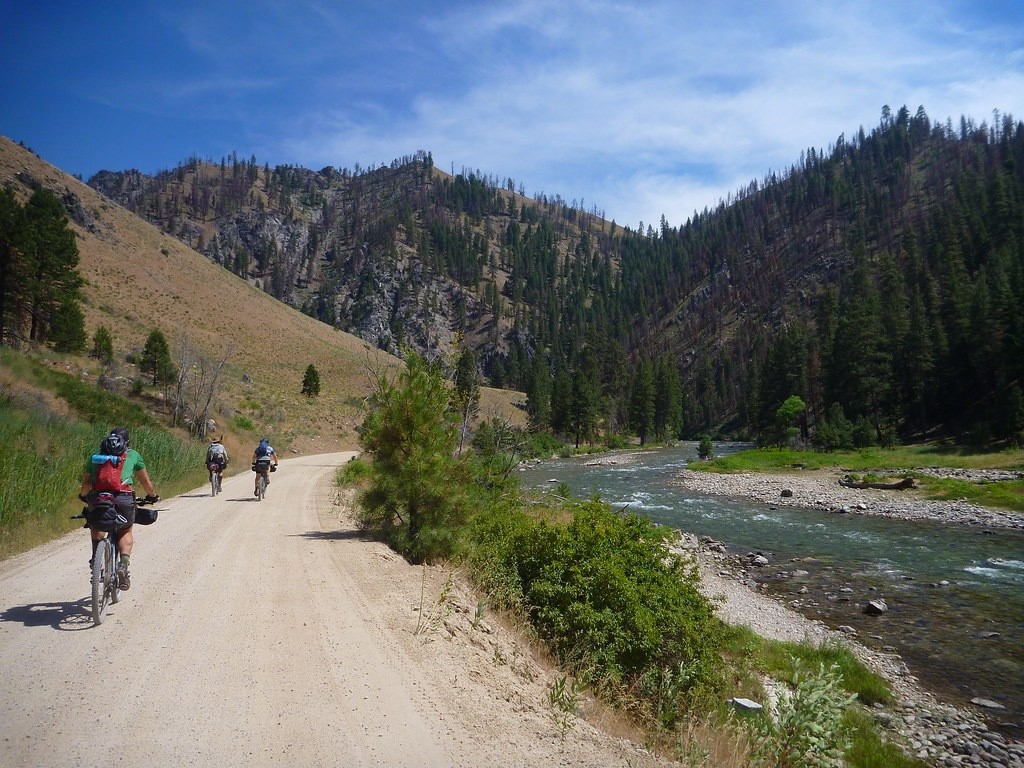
[116,562,130,591]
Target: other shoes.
[266,479,270,483]
[209,476,212,482]
[218,486,221,492]
[254,489,259,496]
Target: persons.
[206,438,229,493]
[252,437,279,498]
[78,430,155,592]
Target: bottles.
[92,454,127,464]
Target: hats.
[212,437,220,442]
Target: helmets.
[101,433,126,455]
[263,439,269,443]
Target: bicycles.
[208,459,229,497]
[250,462,279,501]
[70,495,158,626]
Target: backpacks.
[209,444,220,457]
[259,440,267,454]
[92,451,127,492]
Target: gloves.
[78,494,88,502]
[144,495,159,503]
